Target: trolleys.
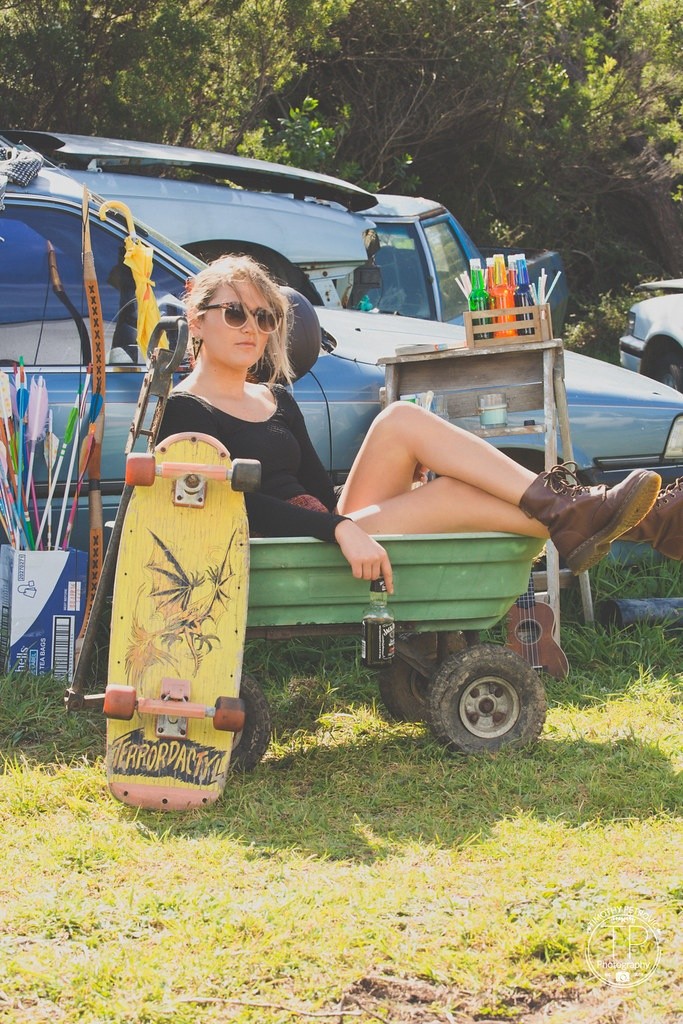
[64,317,549,775]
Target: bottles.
[361,569,396,670]
[468,254,535,339]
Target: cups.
[476,392,508,428]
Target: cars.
[0,140,683,609]
[2,130,379,303]
[620,278,683,391]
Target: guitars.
[503,568,570,681]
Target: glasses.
[200,301,282,335]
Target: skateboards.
[101,431,261,813]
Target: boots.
[518,461,661,576]
[614,476,683,562]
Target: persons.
[155,255,683,593]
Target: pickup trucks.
[284,192,570,344]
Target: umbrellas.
[98,200,172,403]
